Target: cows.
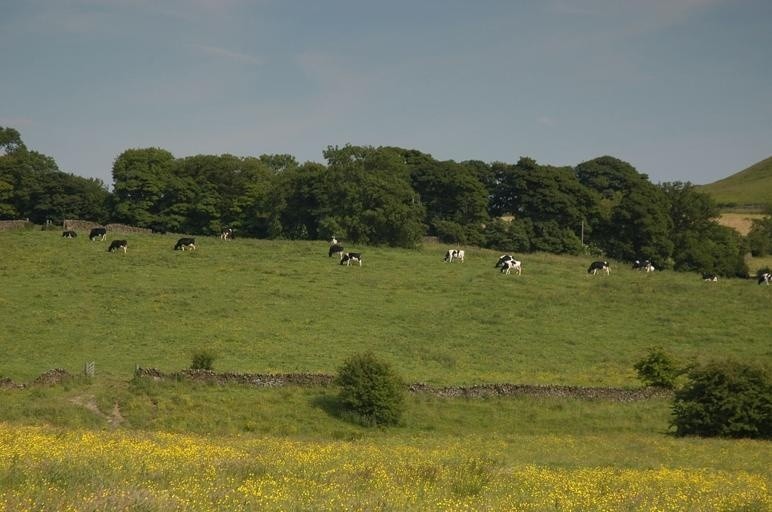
[444,249,465,264]
[495,255,521,274]
[108,239,128,253]
[89,227,106,241]
[174,238,196,251]
[151,225,166,235]
[587,261,612,276]
[632,257,655,273]
[329,236,362,267]
[220,227,235,240]
